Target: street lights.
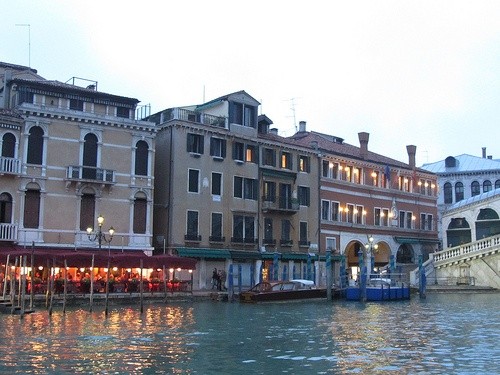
[365,235,378,273]
[87,214,115,275]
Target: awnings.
[310,254,347,261]
[231,249,262,260]
[261,252,282,259]
[281,253,309,260]
[175,248,231,259]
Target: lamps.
[86,214,115,248]
[364,235,379,255]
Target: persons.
[210,267,229,293]
[369,266,392,280]
[0,261,188,296]
[344,268,361,287]
